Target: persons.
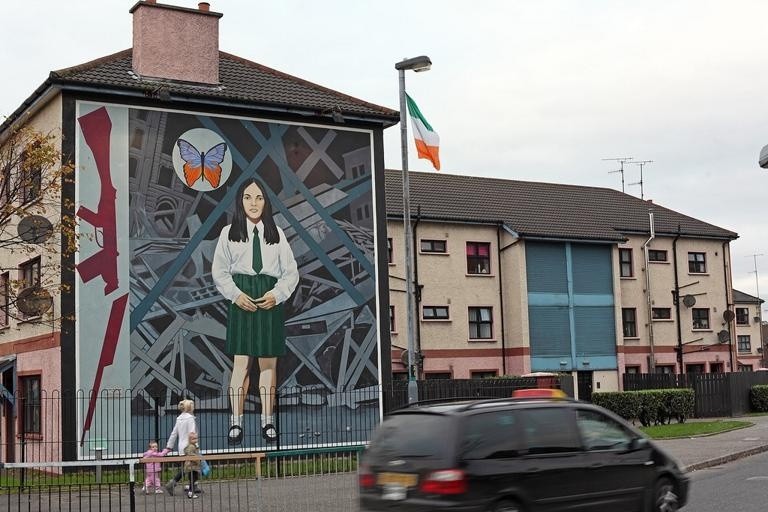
[183,432,202,499]
[164,399,205,495]
[211,177,300,444]
[141,441,170,495]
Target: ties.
[252,226,263,274]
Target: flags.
[406,94,441,172]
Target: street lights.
[395,55,432,407]
[758,144,767,168]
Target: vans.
[358,388,690,512]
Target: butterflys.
[177,138,227,188]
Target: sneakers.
[227,425,243,445]
[142,478,200,499]
[262,424,277,442]
